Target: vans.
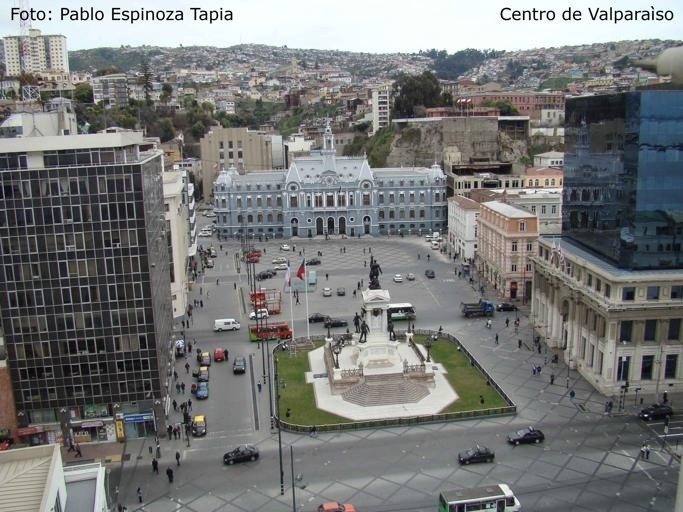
[214,318,241,332]
[425,235,443,250]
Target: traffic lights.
[626,381,629,387]
[625,388,628,392]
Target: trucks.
[460,298,496,318]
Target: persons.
[152,457,160,474]
[519,323,615,417]
[5,439,10,449]
[176,449,180,465]
[166,466,174,483]
[68,438,76,452]
[639,444,647,459]
[144,237,217,443]
[155,440,161,458]
[135,487,144,503]
[0,440,7,450]
[73,442,84,458]
[644,444,652,460]
[219,231,522,343]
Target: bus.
[438,483,522,512]
[390,302,417,321]
[248,320,292,342]
[249,289,282,315]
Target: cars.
[639,404,673,421]
[207,247,218,268]
[191,415,207,438]
[458,444,495,465]
[496,302,518,312]
[223,445,259,465]
[233,356,246,374]
[425,269,435,279]
[308,312,348,328]
[317,501,356,512]
[245,244,290,279]
[393,273,415,282]
[323,287,346,297]
[214,347,225,362]
[248,308,269,320]
[507,425,545,445]
[305,258,322,266]
[196,351,212,399]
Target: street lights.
[423,335,433,363]
[325,318,334,339]
[404,309,414,333]
[332,340,343,369]
[274,358,286,495]
